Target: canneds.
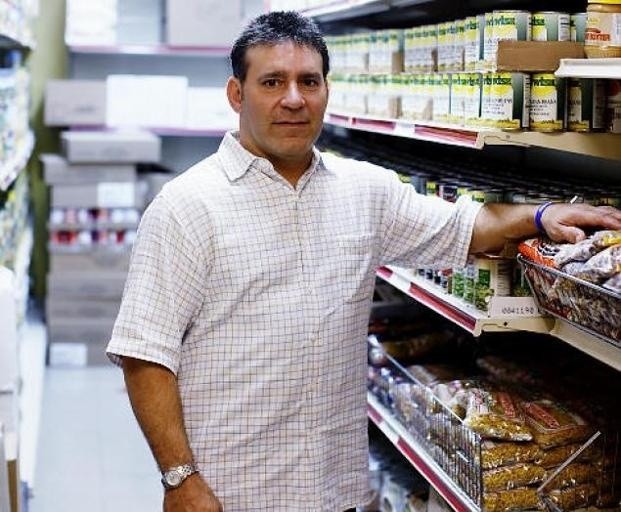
[324,0,621,311]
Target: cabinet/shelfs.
[298,1,620,512]
[0,0,45,512]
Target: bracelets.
[536,200,556,230]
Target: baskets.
[517,253,621,351]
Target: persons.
[104,10,621,512]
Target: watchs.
[160,459,200,491]
